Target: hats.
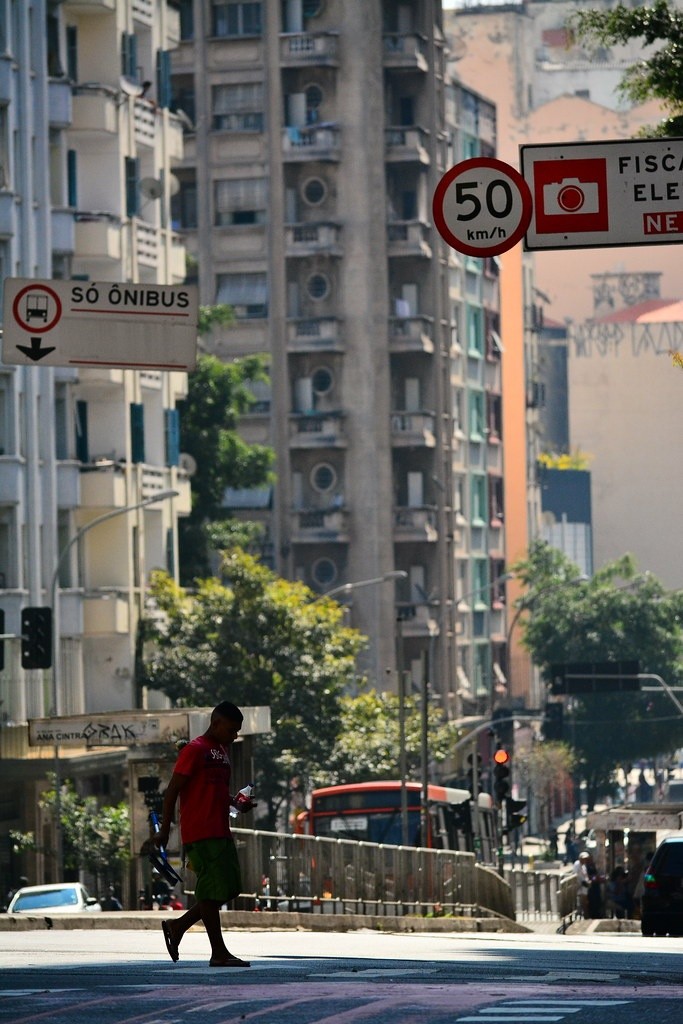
[579,852,589,859]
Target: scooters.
[138,885,175,911]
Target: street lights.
[52,487,182,886]
[447,568,517,721]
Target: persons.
[548,821,654,919]
[3,877,29,910]
[99,874,287,912]
[152,700,257,968]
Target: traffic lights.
[493,746,509,796]
[466,751,482,798]
[501,796,528,830]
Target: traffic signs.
[3,277,199,373]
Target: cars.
[637,837,683,937]
[0,881,103,915]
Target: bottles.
[229,782,254,818]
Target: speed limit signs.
[432,155,534,258]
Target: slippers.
[209,955,250,967]
[162,919,181,964]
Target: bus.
[295,780,492,916]
